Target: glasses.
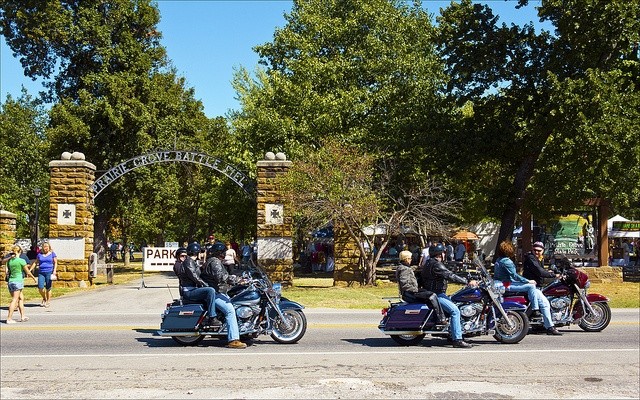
[534,248,542,252]
[180,255,187,258]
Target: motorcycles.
[452,255,610,335]
[374,245,528,347]
[152,250,306,347]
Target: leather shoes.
[546,327,562,335]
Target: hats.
[208,235,214,239]
[534,242,544,248]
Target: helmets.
[429,243,448,258]
[186,243,200,256]
[399,251,413,263]
[212,242,228,256]
[176,248,186,254]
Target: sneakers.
[453,340,473,348]
[533,310,543,317]
[226,340,247,349]
[21,317,29,322]
[208,317,223,326]
[6,319,17,324]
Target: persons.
[27,242,58,307]
[419,243,479,348]
[5,246,37,324]
[395,250,449,324]
[180,244,222,324]
[26,246,38,278]
[374,238,475,272]
[220,238,241,274]
[204,235,217,264]
[521,241,565,337]
[494,240,542,315]
[608,238,640,268]
[201,241,247,348]
[238,241,252,266]
[2,245,31,311]
[174,247,189,302]
[107,239,137,262]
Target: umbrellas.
[450,230,478,241]
